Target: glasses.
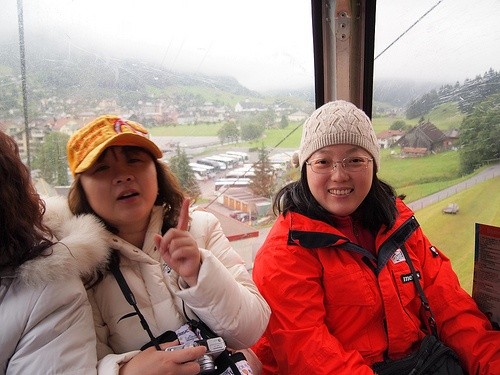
[303,156,373,174]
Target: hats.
[292,100,380,172]
[67,116,163,173]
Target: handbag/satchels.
[368,336,465,375]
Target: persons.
[248,100,500,375]
[67,115,271,374]
[0,130,110,375]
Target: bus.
[188,161,216,180]
[225,163,283,178]
[267,151,298,169]
[197,151,250,173]
[217,178,250,182]
[215,181,250,191]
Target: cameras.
[165,337,226,373]
